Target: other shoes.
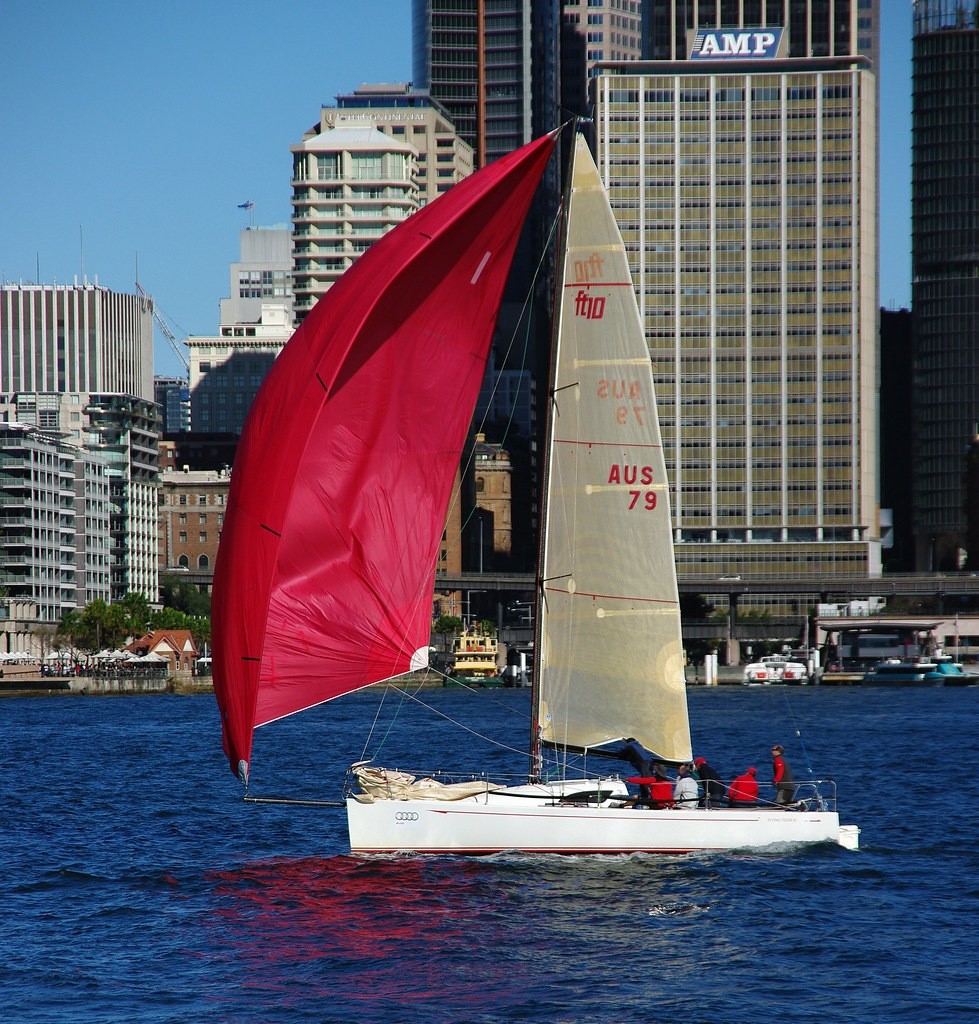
[610,802,624,808]
[799,801,808,812]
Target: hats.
[694,757,705,764]
[771,745,784,754]
[745,768,757,773]
[653,764,667,777]
[626,737,636,743]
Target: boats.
[438,622,505,690]
[860,641,979,690]
[741,646,824,686]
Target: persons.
[879,652,922,665]
[693,757,726,808]
[727,766,759,808]
[620,738,654,808]
[770,744,809,812]
[671,764,700,810]
[1,661,149,677]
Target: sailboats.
[205,116,864,852]
